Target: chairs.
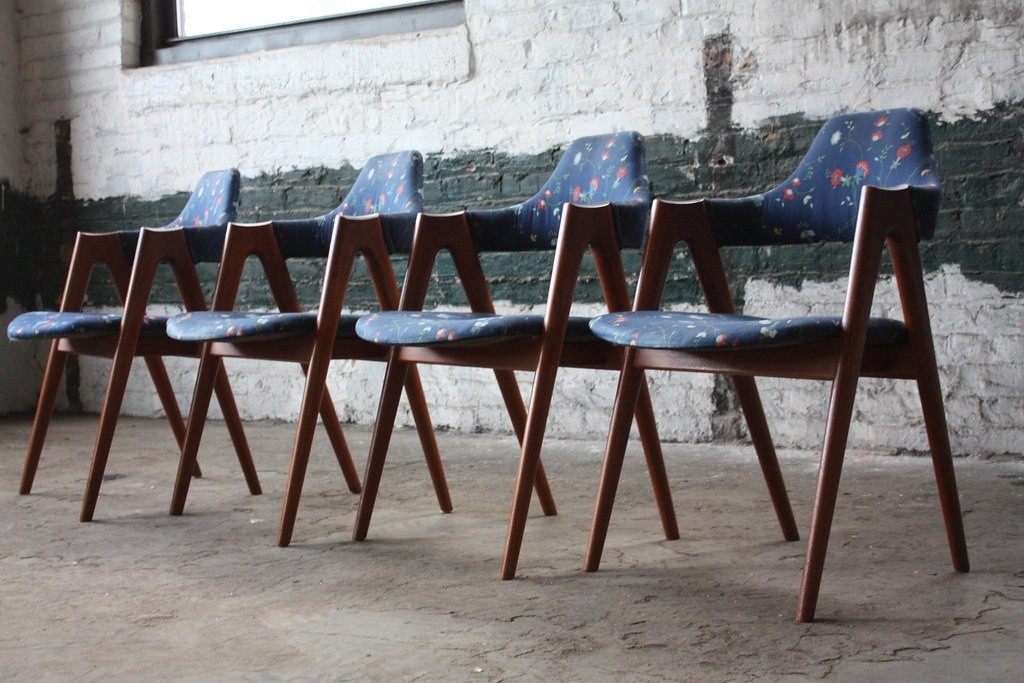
[6,168,263,522]
[169,148,454,547]
[581,107,970,622]
[352,130,679,581]
